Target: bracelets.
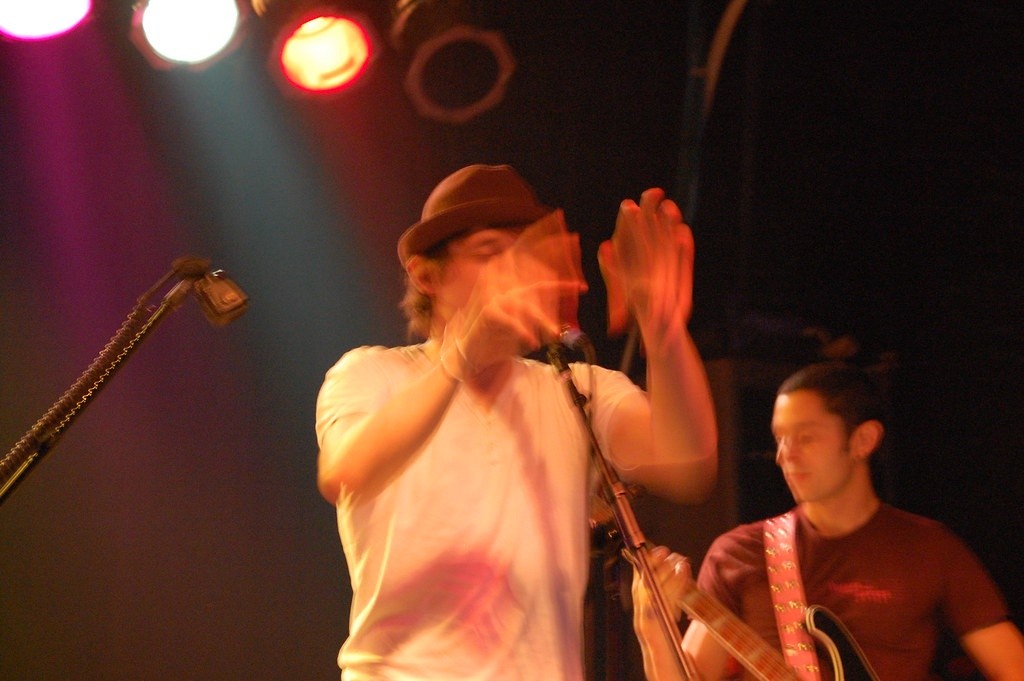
[442,337,481,381]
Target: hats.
[398,164,548,271]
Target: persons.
[315,164,717,681]
[631,339,1024,681]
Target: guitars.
[587,492,884,681]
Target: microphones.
[553,319,590,356]
[173,255,249,328]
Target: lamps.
[0,0,523,129]
